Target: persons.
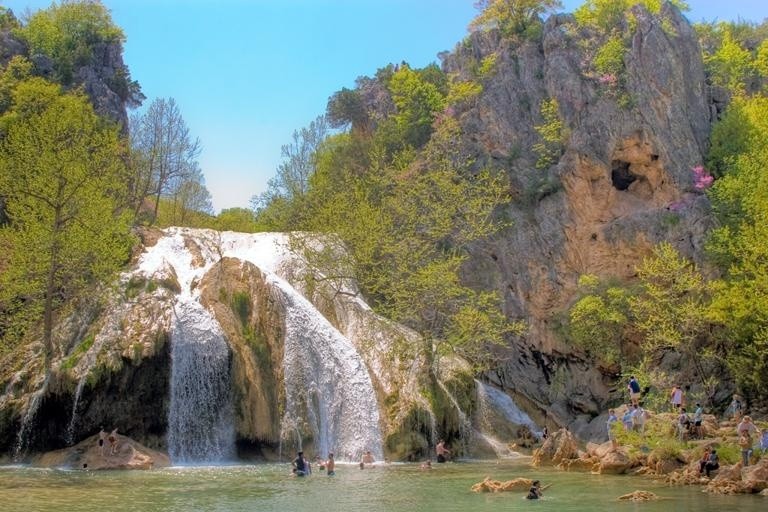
[436,439,452,464]
[421,459,432,470]
[542,425,548,439]
[360,450,377,470]
[526,479,552,499]
[291,451,336,479]
[606,374,647,453]
[97,426,120,457]
[670,383,768,479]
